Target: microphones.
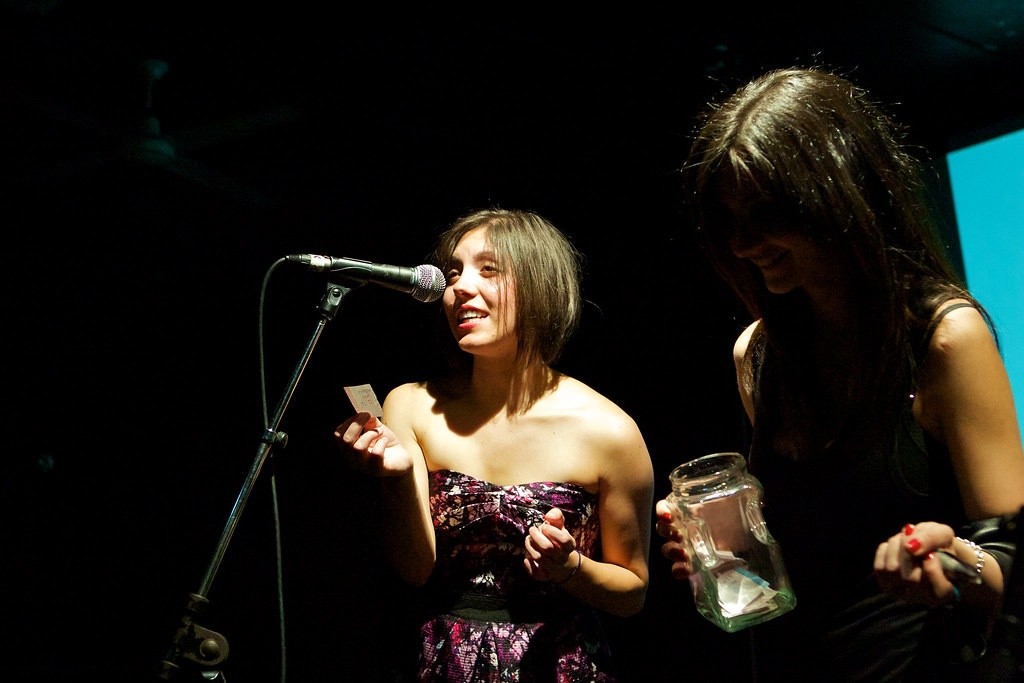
[285,253,446,302]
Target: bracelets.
[550,550,581,584]
[953,537,984,574]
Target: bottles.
[670,450,797,632]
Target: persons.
[335,210,654,683]
[656,69,1024,683]
